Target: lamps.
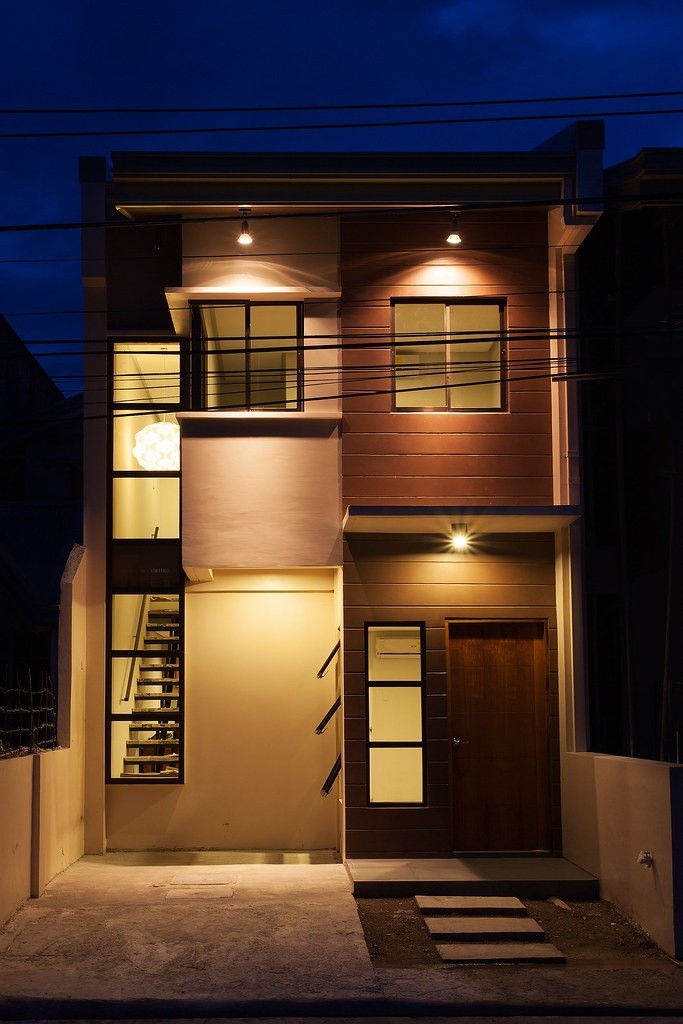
[445,215,462,244]
[238,220,252,244]
